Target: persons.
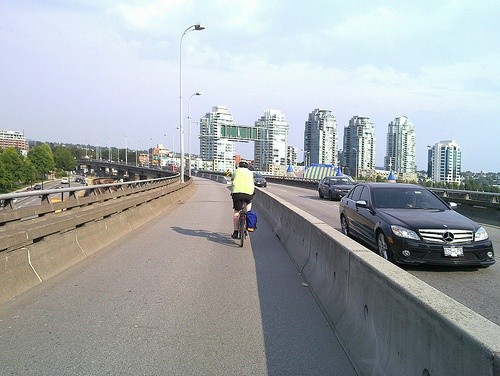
[406,191,416,204]
[230,162,254,239]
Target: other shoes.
[232,233,238,239]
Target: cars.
[252,172,267,188]
[317,176,358,201]
[338,182,496,269]
[34,185,41,190]
[39,195,43,198]
[75,178,86,184]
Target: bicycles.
[230,186,256,248]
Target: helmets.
[239,161,249,165]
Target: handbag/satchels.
[246,211,257,229]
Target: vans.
[61,177,69,184]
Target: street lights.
[352,147,358,181]
[186,92,201,178]
[106,116,192,173]
[178,23,205,184]
